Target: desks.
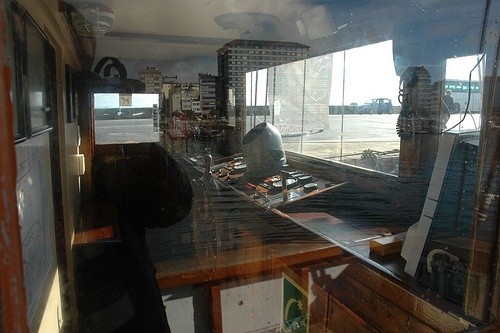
[150,183,414,333]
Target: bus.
[434,79,482,113]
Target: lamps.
[71,2,116,39]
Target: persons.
[55,71,191,333]
